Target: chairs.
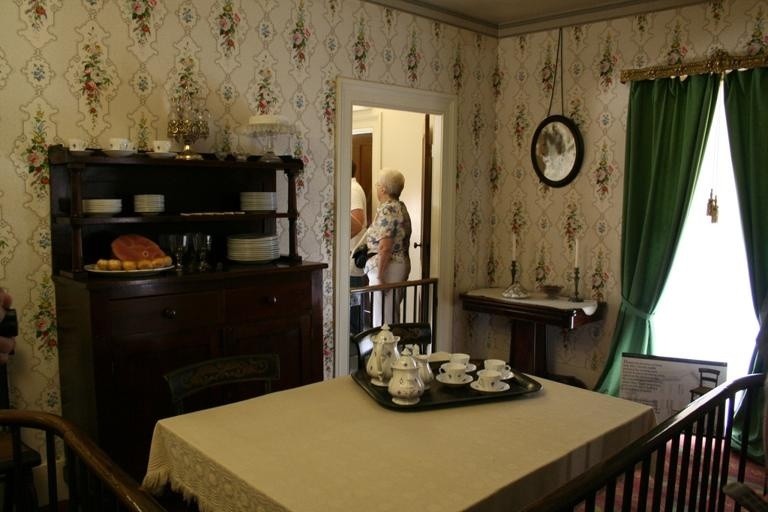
[350,319,433,366]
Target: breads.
[96,257,174,270]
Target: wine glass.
[168,232,213,273]
[542,284,563,300]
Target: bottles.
[415,353,434,391]
[501,272,532,299]
[387,348,426,405]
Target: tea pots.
[367,323,401,388]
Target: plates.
[84,263,175,276]
[240,191,278,213]
[226,233,281,264]
[133,194,165,215]
[81,198,123,217]
[68,149,178,158]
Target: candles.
[574,238,579,268]
[512,231,517,261]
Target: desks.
[460,286,607,388]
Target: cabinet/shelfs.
[46,142,330,512]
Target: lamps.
[243,111,296,163]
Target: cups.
[63,137,174,152]
[435,353,516,394]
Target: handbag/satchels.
[352,242,367,269]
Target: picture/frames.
[529,115,585,189]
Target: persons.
[365,167,412,329]
[351,159,367,344]
